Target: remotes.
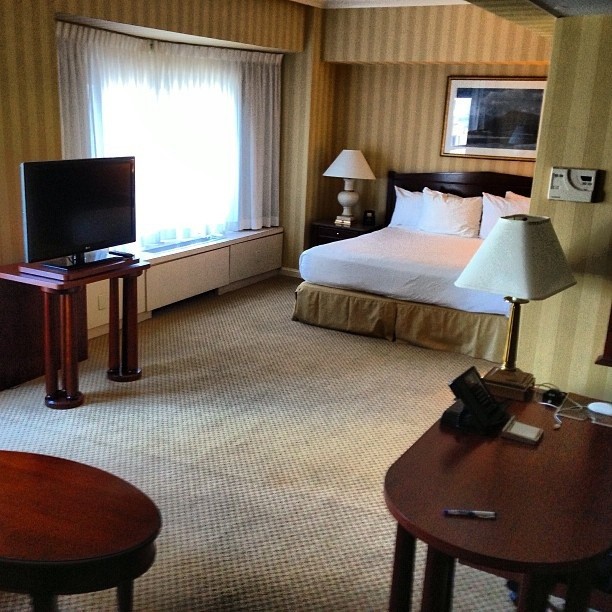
[109,250,135,258]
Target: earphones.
[553,415,562,430]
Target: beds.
[291,172,534,364]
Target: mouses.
[587,401,612,417]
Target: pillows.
[503,188,531,207]
[388,185,425,233]
[478,193,531,239]
[418,186,482,238]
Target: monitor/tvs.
[20,155,136,271]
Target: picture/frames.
[440,73,547,164]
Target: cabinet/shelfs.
[146,233,283,312]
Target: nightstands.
[308,219,383,247]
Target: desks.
[0,254,151,409]
[384,385,610,612]
[1,450,162,612]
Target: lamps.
[323,149,376,227]
[453,216,578,402]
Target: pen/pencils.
[443,509,496,519]
